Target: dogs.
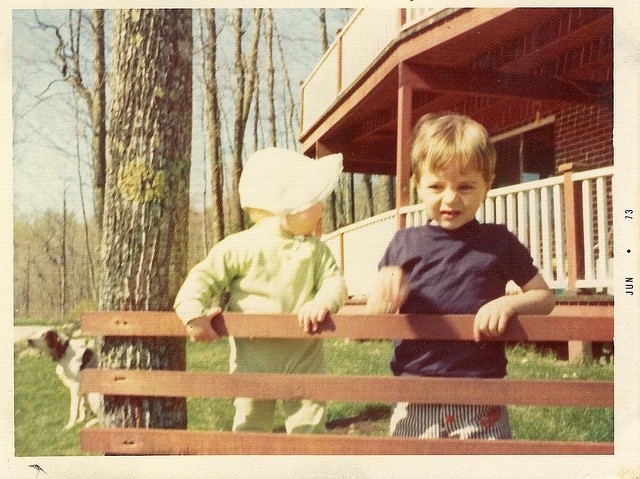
[27,331,104,430]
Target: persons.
[172,148,349,434]
[363,113,555,440]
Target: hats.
[238,148,345,215]
